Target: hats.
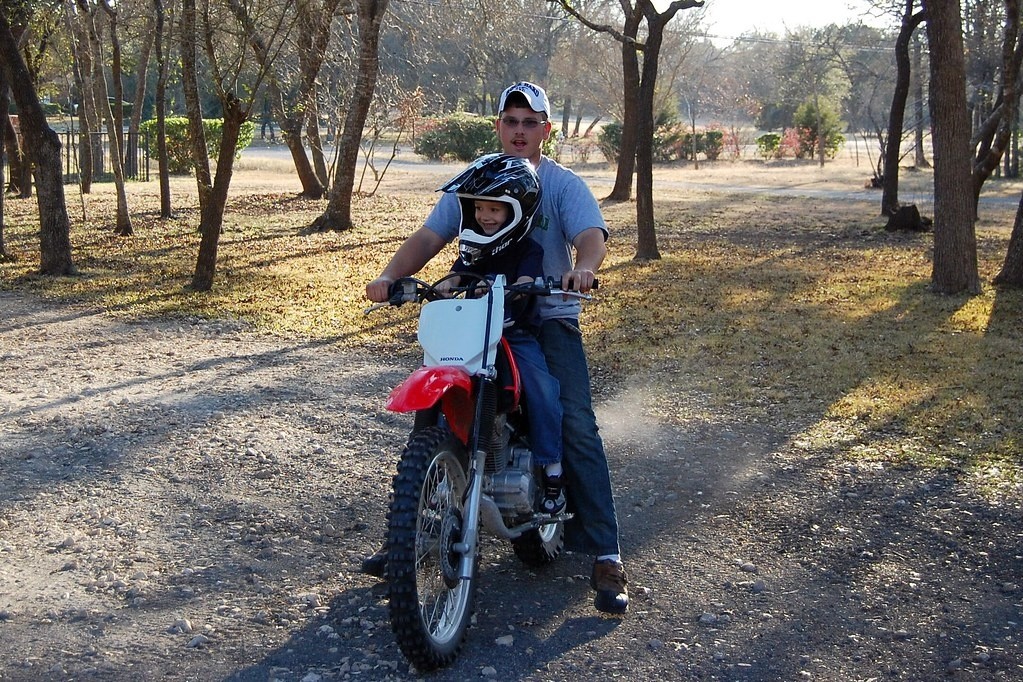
[496,80,552,118]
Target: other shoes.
[542,463,564,491]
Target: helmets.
[435,153,542,268]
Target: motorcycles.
[360,274,601,670]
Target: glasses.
[498,115,548,129]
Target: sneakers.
[361,540,388,576]
[590,558,630,614]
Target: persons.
[433,155,566,516]
[361,83,630,614]
